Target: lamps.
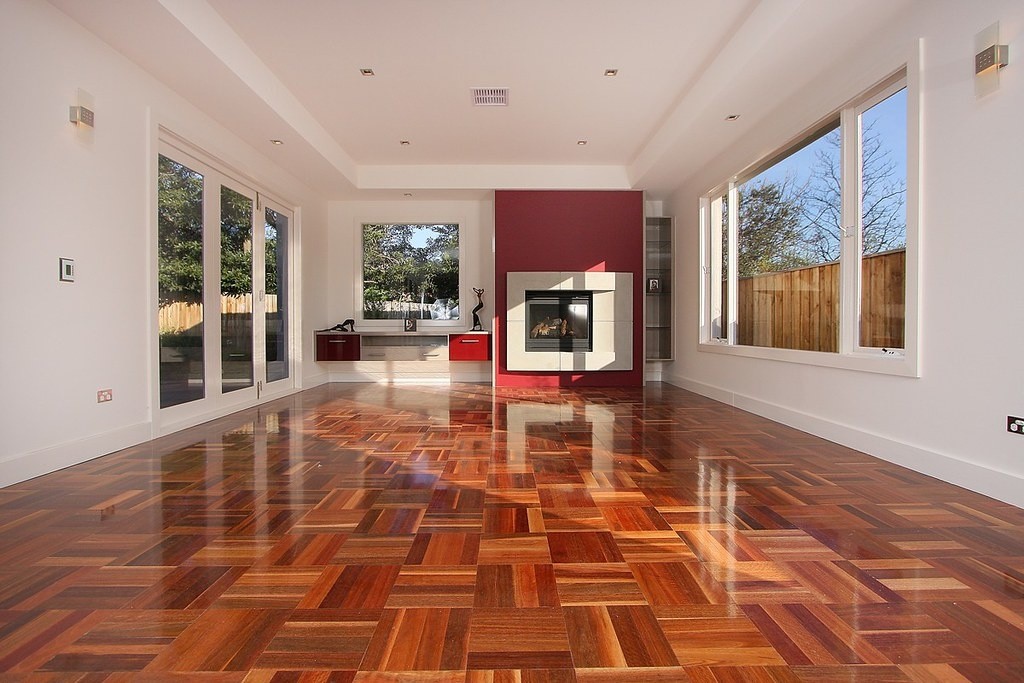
[70,106,95,130]
[974,43,1009,75]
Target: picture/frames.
[647,277,660,293]
[404,318,417,332]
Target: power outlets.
[97,389,113,404]
[1007,415,1024,436]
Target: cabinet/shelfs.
[314,328,493,364]
[646,215,677,362]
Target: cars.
[429,299,454,319]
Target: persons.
[471,288,484,330]
